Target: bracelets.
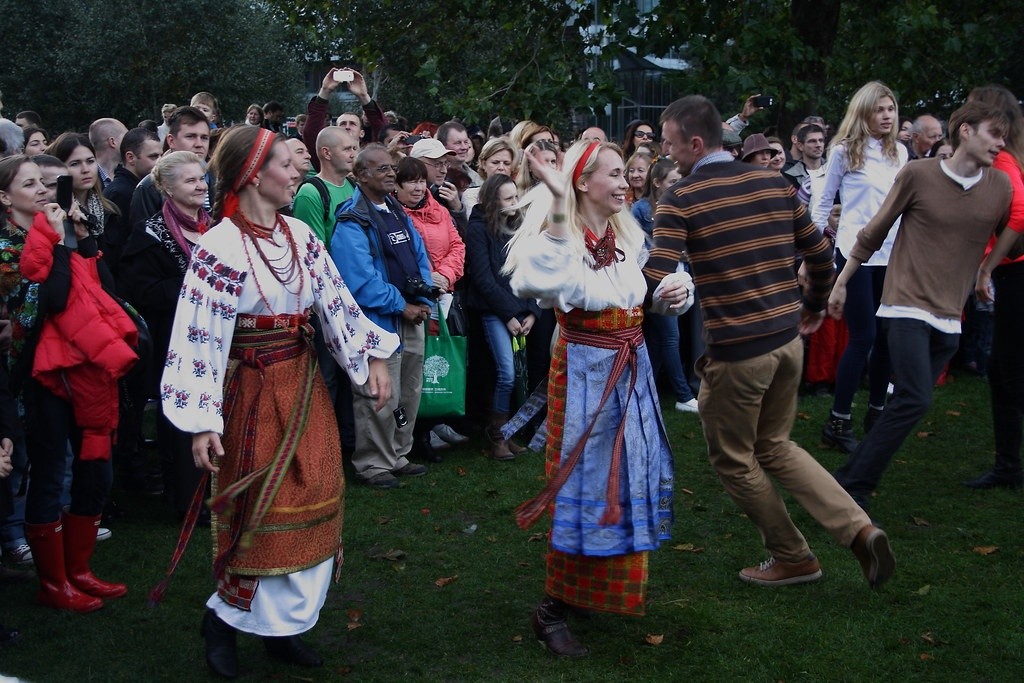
[549,213,566,225]
[980,269,991,278]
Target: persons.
[500,139,695,659]
[0,92,225,614]
[826,101,1023,518]
[960,86,1024,491]
[641,95,897,587]
[796,81,909,457]
[161,126,403,680]
[623,93,996,413]
[244,102,284,133]
[578,126,609,142]
[277,134,311,217]
[286,66,568,490]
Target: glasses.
[425,160,451,168]
[376,166,400,175]
[634,131,656,139]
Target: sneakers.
[739,553,823,587]
[850,524,897,591]
[8,544,33,564]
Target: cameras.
[393,405,408,428]
[403,273,441,301]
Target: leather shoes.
[200,609,241,677]
[262,634,322,668]
[821,413,860,454]
[532,596,586,659]
[864,407,883,433]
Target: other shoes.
[429,430,449,450]
[96,527,111,540]
[392,462,428,477]
[815,383,833,399]
[408,431,443,463]
[433,424,470,444]
[963,452,1023,488]
[368,472,399,489]
[675,396,699,413]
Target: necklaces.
[233,207,304,333]
[583,219,626,271]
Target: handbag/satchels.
[513,334,530,409]
[417,303,468,418]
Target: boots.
[485,410,528,461]
[24,519,104,615]
[63,511,128,600]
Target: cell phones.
[755,95,775,106]
[332,70,354,82]
[56,175,73,212]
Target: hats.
[742,133,778,161]
[410,139,457,159]
[721,129,743,146]
